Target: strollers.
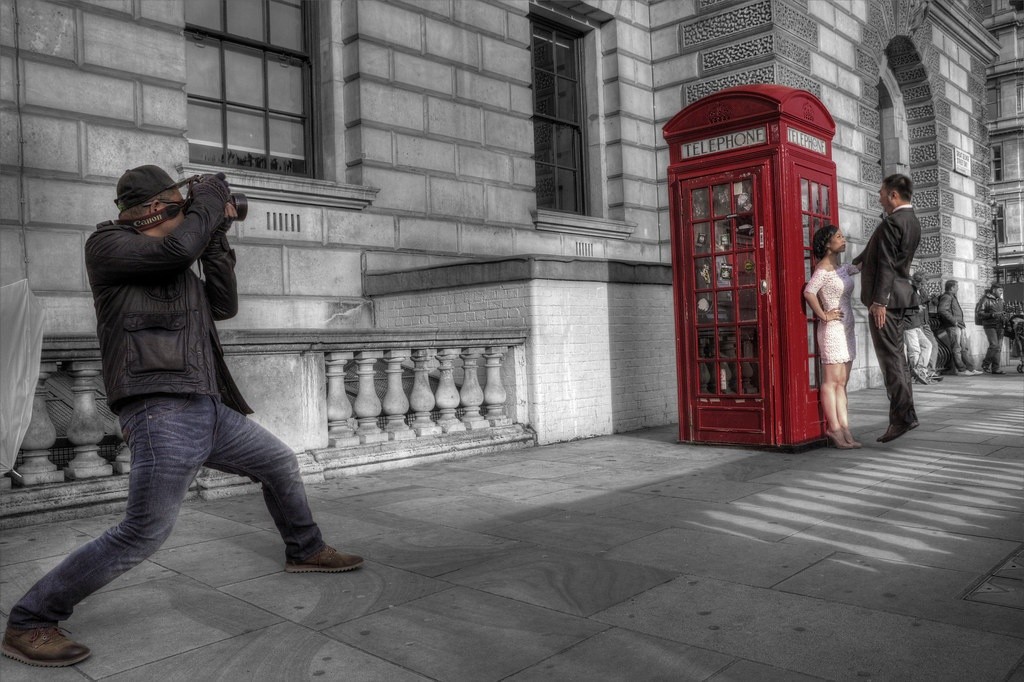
[1006,314,1024,372]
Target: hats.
[114,165,196,212]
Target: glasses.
[142,199,191,210]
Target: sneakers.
[285,542,365,572]
[0,625,92,668]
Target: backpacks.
[975,295,989,325]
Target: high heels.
[824,426,853,450]
[838,426,862,449]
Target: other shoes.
[971,370,983,375]
[982,363,990,373]
[958,369,975,376]
[927,370,943,382]
[992,369,1006,374]
[910,364,929,384]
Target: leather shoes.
[876,418,920,443]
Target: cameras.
[181,173,248,222]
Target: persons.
[2,164,366,668]
[852,174,919,444]
[979,284,1007,374]
[938,280,983,376]
[902,271,943,384]
[804,225,864,450]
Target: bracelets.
[820,313,828,323]
